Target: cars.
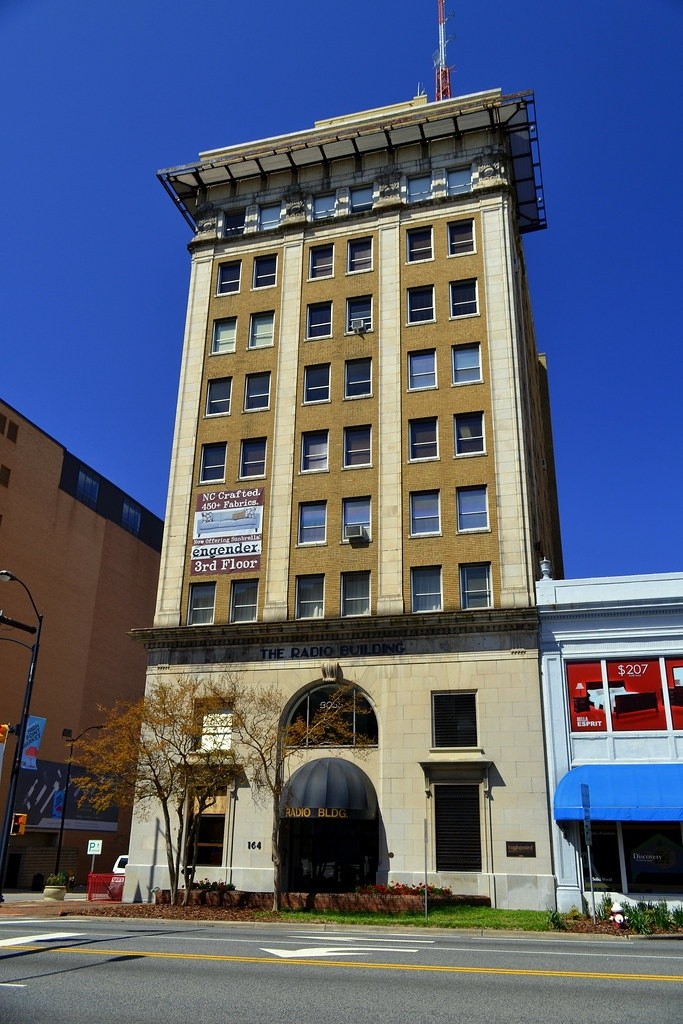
[112,855,129,873]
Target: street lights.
[0,571,45,902]
[53,725,112,875]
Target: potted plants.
[42,873,67,901]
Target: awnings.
[554,764,683,822]
[278,757,379,819]
